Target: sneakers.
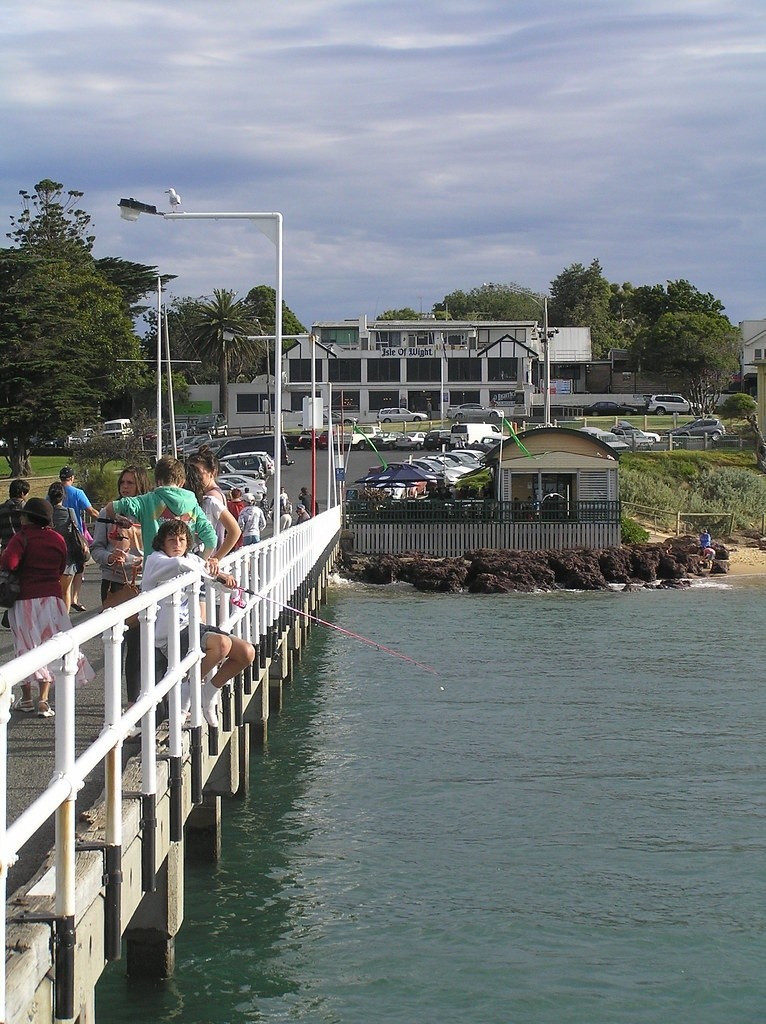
[181,685,191,723]
[201,685,219,728]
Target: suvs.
[665,418,726,442]
[643,393,702,415]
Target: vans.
[214,435,289,465]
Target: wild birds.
[164,188,182,213]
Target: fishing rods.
[97,516,141,542]
[214,577,449,682]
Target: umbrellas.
[353,466,444,487]
[426,401,432,420]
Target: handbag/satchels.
[0,572,20,607]
[102,562,141,629]
[68,508,90,562]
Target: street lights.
[117,197,284,535]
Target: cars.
[368,449,487,484]
[318,423,511,453]
[611,426,652,451]
[217,480,244,497]
[218,454,268,478]
[376,407,428,422]
[577,427,630,450]
[219,475,268,497]
[584,401,637,415]
[220,451,275,476]
[640,429,661,444]
[297,409,359,425]
[445,403,504,418]
[220,462,260,479]
[12,410,241,460]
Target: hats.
[296,504,305,510]
[239,493,255,502]
[13,498,53,522]
[59,465,74,478]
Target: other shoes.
[1,611,11,630]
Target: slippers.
[71,603,86,611]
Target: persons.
[699,529,716,569]
[427,482,495,518]
[0,446,320,727]
[490,399,498,408]
[513,496,535,521]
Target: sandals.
[38,701,56,717]
[11,697,34,712]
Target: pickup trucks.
[284,430,320,450]
